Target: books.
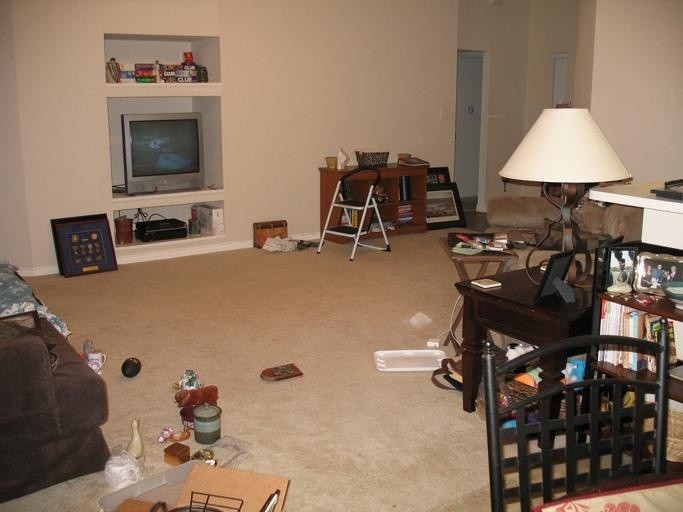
[597,297,680,372]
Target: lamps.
[499,109,633,287]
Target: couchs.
[0,259,112,501]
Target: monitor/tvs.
[121,112,205,197]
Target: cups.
[191,402,222,444]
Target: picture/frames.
[605,246,639,293]
[428,167,451,184]
[426,182,466,231]
[633,252,683,297]
[534,250,576,304]
[51,212,119,278]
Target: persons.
[642,260,653,285]
[615,260,629,288]
[664,261,679,288]
[650,263,665,288]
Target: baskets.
[644,409,682,463]
[483,369,586,432]
[354,150,389,168]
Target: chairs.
[316,166,392,262]
[480,318,669,512]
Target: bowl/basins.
[662,280,682,310]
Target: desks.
[454,261,604,449]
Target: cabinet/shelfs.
[317,160,429,245]
[592,290,683,471]
